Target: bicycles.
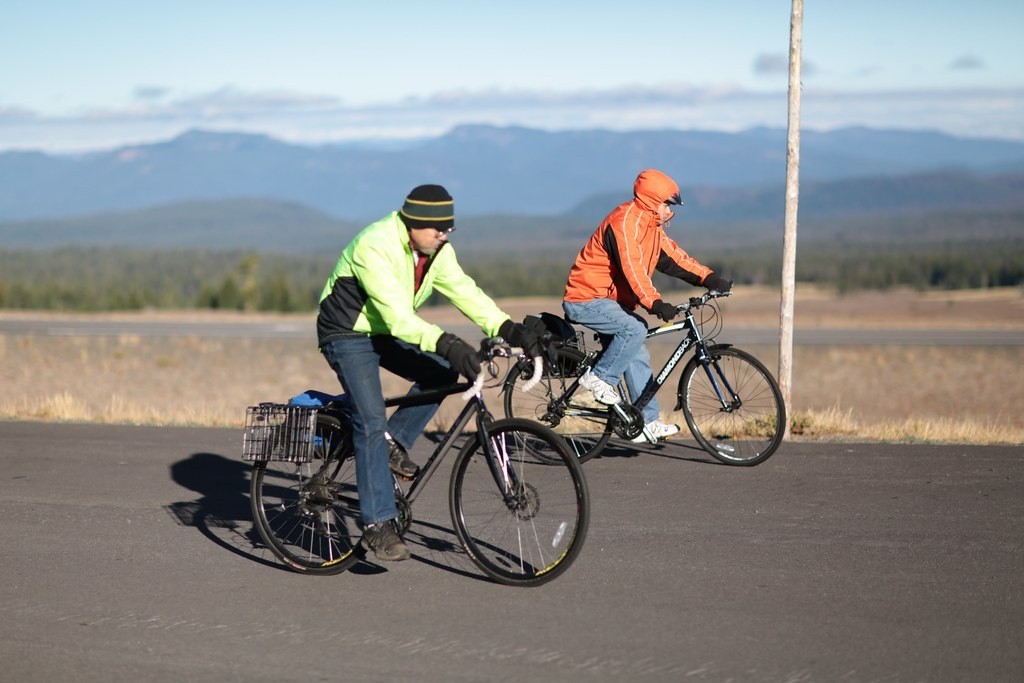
[497,278,785,469]
[241,318,591,592]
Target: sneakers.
[577,366,622,405]
[632,420,681,444]
[362,520,411,561]
[383,438,420,477]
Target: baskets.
[522,329,586,378]
[242,403,318,464]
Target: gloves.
[649,299,678,323]
[500,318,555,355]
[703,272,730,296]
[436,331,481,384]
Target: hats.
[401,184,454,228]
[664,191,684,206]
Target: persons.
[560,168,735,447]
[316,184,548,571]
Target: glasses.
[433,226,456,238]
